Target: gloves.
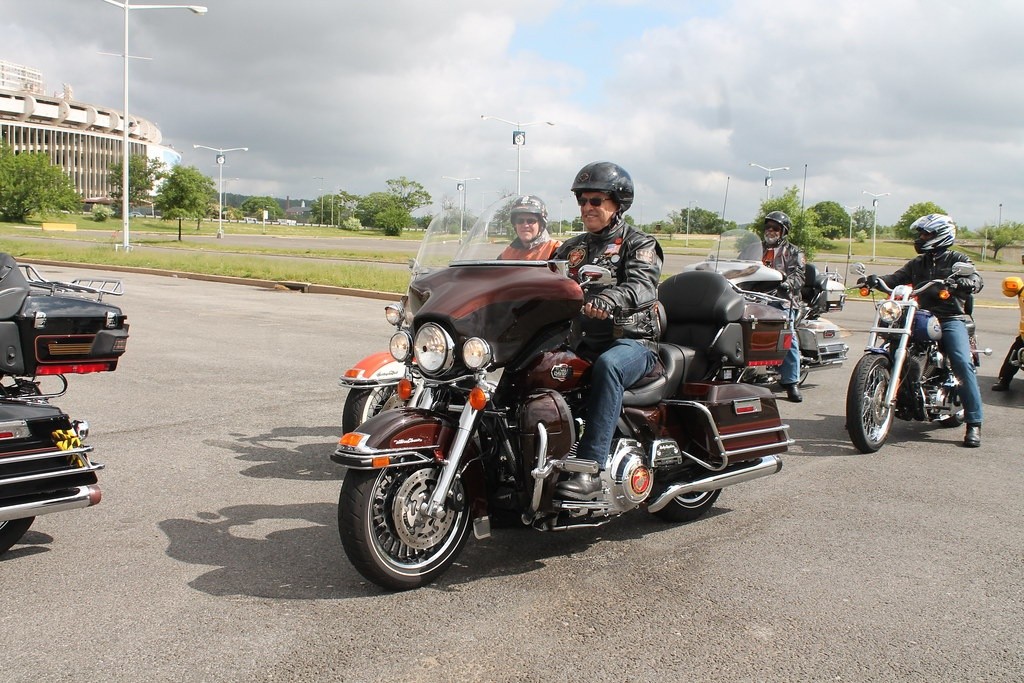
[957,277,976,293]
[779,282,790,294]
[857,274,878,287]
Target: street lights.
[862,191,890,261]
[441,177,480,233]
[329,199,340,225]
[685,200,697,247]
[102,0,208,251]
[999,204,1003,228]
[193,145,248,238]
[318,189,333,225]
[313,177,324,224]
[750,163,790,201]
[984,226,996,262]
[844,206,859,258]
[482,116,554,194]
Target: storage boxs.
[820,277,846,303]
[798,318,843,365]
[685,382,787,461]
[743,300,792,366]
[24,293,130,377]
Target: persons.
[496,196,563,260]
[844,214,984,448]
[548,160,665,503]
[735,210,806,403]
[992,330,1022,391]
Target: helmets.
[511,196,548,233]
[570,161,634,219]
[913,214,956,254]
[765,211,791,235]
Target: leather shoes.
[555,470,604,501]
[787,383,803,401]
[992,380,1009,390]
[965,427,981,446]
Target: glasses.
[513,218,539,225]
[578,197,613,206]
[763,223,782,232]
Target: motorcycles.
[846,262,1024,455]
[1,249,131,554]
[330,194,849,592]
